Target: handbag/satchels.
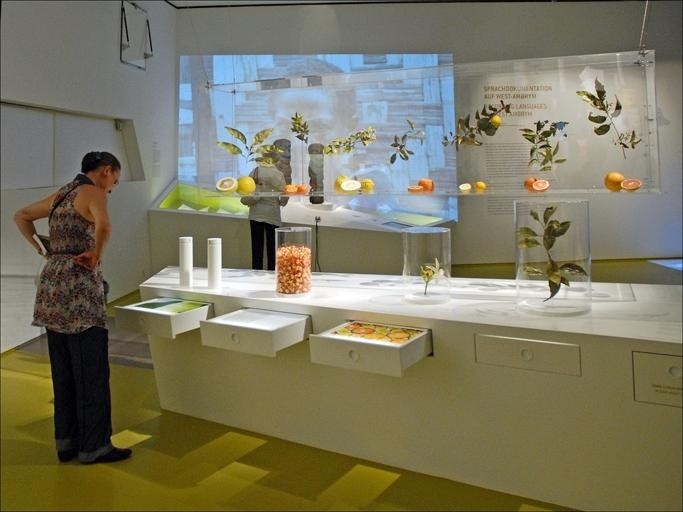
[241,167,261,206]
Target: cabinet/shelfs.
[112,262,682,512]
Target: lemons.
[216,176,238,193]
[336,175,374,194]
[491,115,501,129]
[458,181,486,193]
[336,322,420,344]
[238,177,256,193]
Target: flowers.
[418,258,444,296]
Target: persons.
[13,150,133,465]
[241,152,289,271]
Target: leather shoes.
[57,448,131,462]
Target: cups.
[399,227,456,303]
[273,225,314,295]
[508,195,591,313]
[206,236,223,291]
[177,235,194,288]
